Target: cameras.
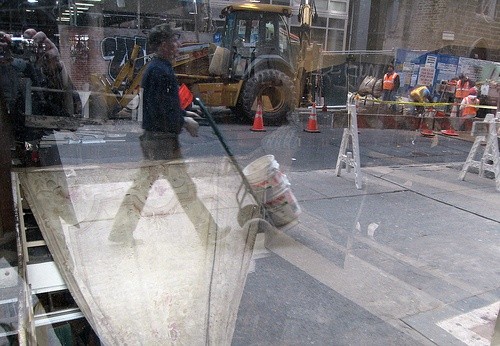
[0,34,46,69]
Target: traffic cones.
[248,97,269,131]
[420,105,437,138]
[440,100,461,138]
[354,94,360,113]
[304,101,323,134]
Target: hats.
[468,87,478,93]
[146,24,182,54]
[387,64,394,70]
[423,89,430,94]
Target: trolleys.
[179,96,303,252]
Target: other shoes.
[108,235,142,245]
[202,225,231,245]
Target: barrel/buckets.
[242,154,302,226]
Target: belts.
[145,127,177,134]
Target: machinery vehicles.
[85,4,325,126]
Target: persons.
[0,27,104,346]
[449,73,480,123]
[380,63,401,103]
[410,85,433,117]
[107,21,232,248]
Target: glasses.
[162,33,181,41]
